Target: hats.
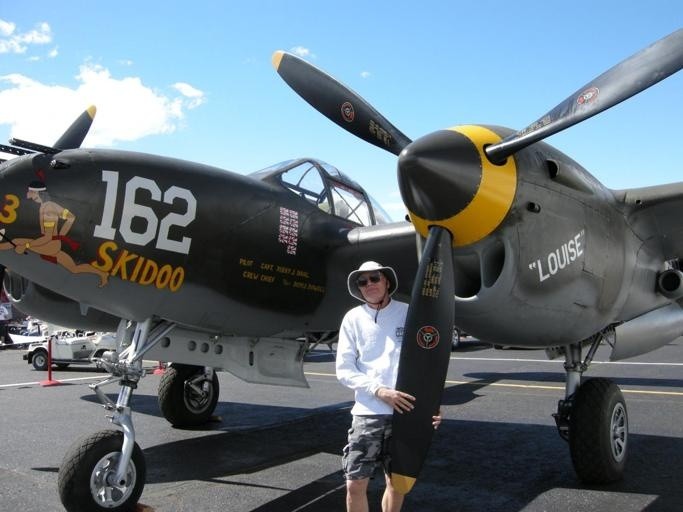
[347,261,399,302]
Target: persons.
[334,261,443,512]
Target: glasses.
[357,273,383,286]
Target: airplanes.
[1,29,682,511]
